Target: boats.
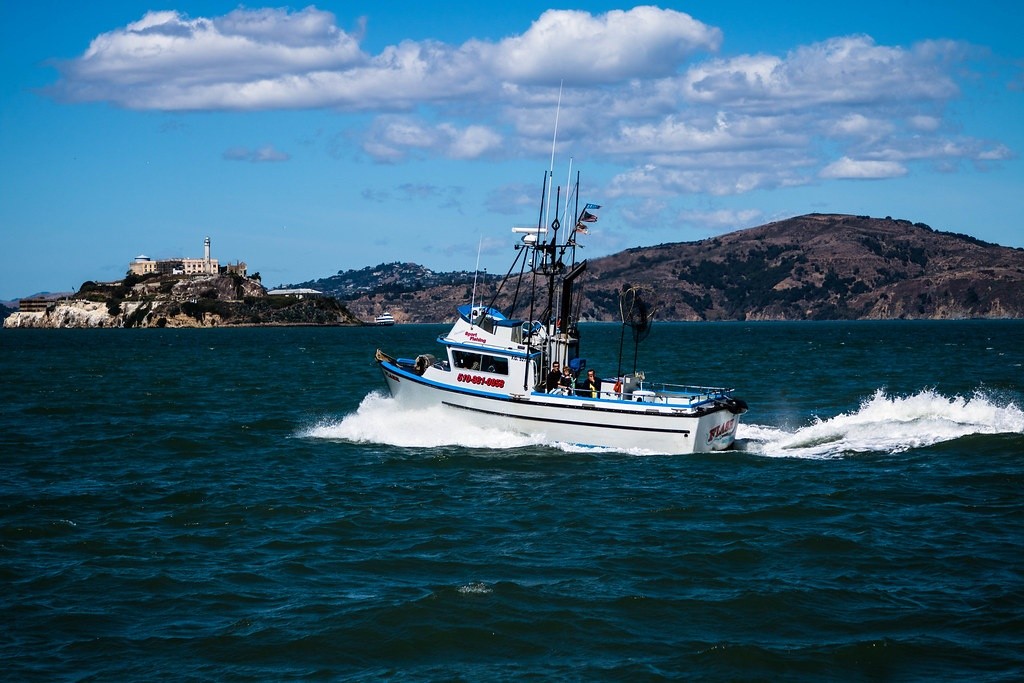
[375,309,395,326]
[374,76,750,458]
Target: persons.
[547,361,563,394]
[557,367,577,396]
[583,369,603,399]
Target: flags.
[586,204,602,209]
[581,210,597,222]
[573,222,591,236]
